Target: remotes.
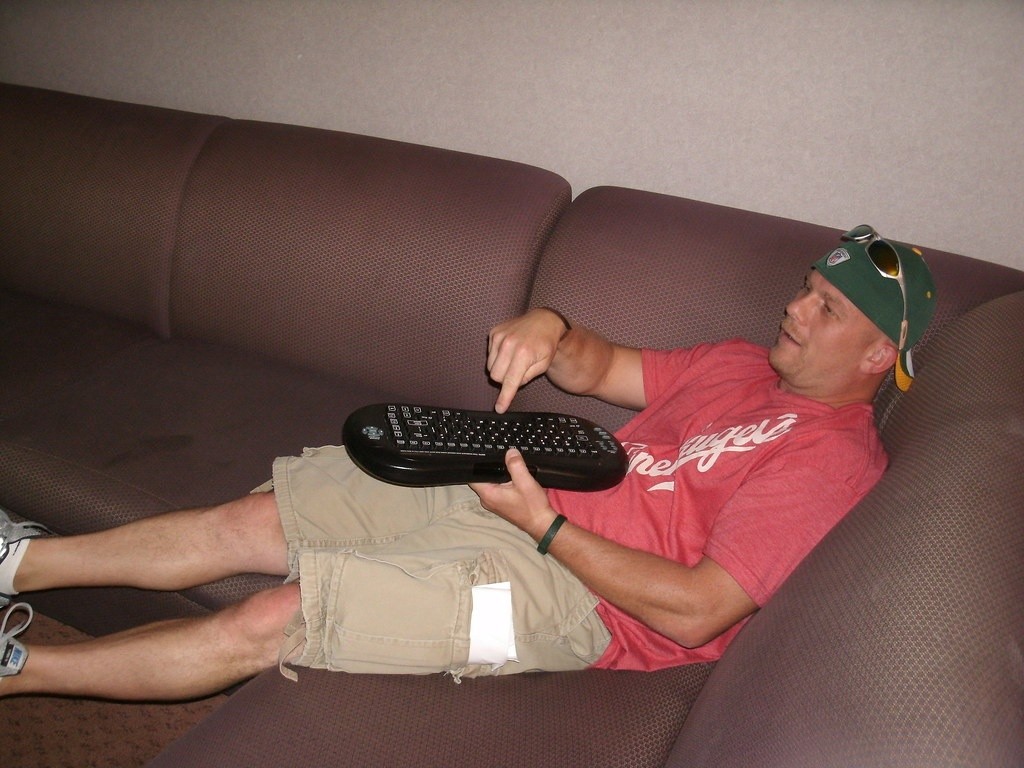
[344,404,629,492]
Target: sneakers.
[0,510,37,611]
[0,629,27,681]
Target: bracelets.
[534,305,573,344]
[536,513,568,556]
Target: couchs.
[0,83,1023,768]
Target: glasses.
[841,222,906,351]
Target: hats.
[808,240,939,392]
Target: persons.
[1,224,941,703]
